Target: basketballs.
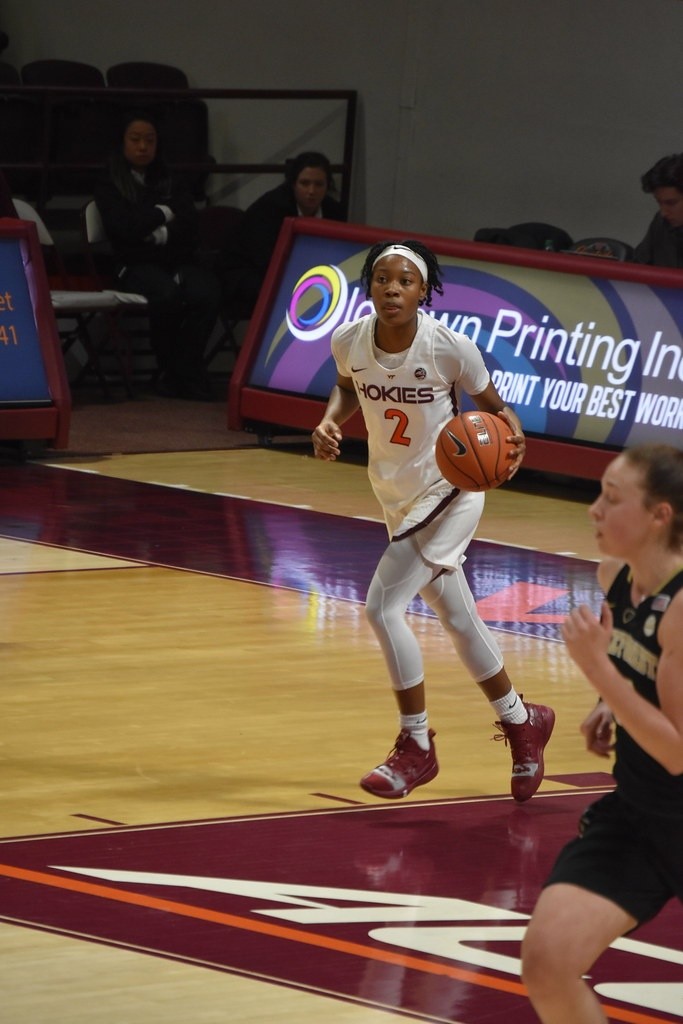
[435,411,518,492]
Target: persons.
[628,154,683,266]
[95,111,351,395]
[310,238,555,805]
[520,444,682,1024]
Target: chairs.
[0,34,635,403]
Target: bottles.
[544,240,553,252]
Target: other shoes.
[152,366,212,401]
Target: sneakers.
[359,729,439,799]
[495,702,556,802]
